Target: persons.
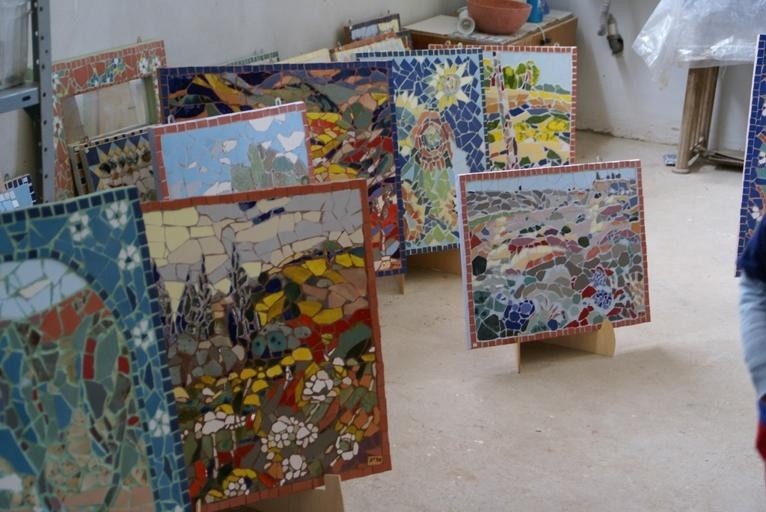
[737,213,766,461]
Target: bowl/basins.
[467,0,533,36]
